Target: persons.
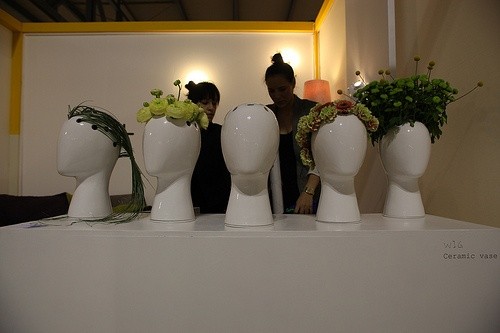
[264,53,323,216]
[185,80,231,215]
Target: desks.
[0,211,500,333]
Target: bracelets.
[302,186,315,195]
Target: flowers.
[295,98,380,172]
[351,57,484,146]
[137,80,210,132]
